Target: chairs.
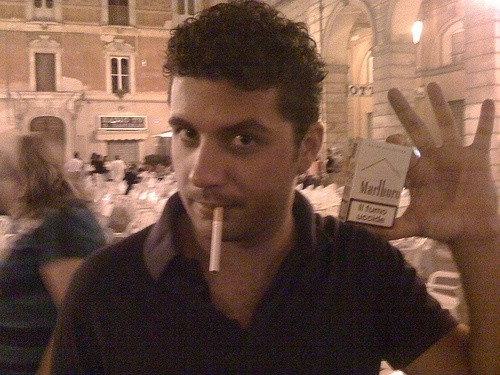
[0,172,463,323]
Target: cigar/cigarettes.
[209,205,224,272]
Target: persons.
[90,154,105,173]
[64,152,83,181]
[302,160,320,189]
[89,152,98,163]
[110,154,127,183]
[123,163,138,196]
[325,148,338,174]
[50,1,500,375]
[102,156,112,173]
[1,133,109,375]
